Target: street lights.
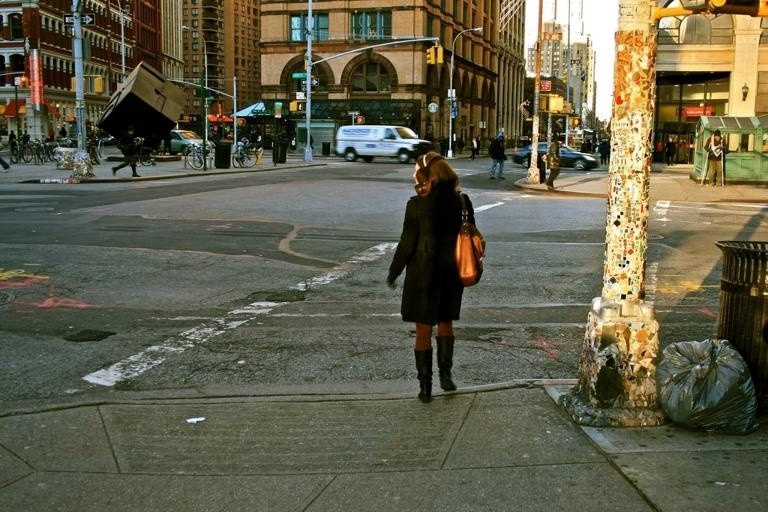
[447,27,483,159]
[181,25,208,141]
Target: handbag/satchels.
[454,220,485,287]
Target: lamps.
[742,82,749,101]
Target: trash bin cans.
[215,143,231,169]
[273,140,287,163]
[322,142,330,155]
[714,240,768,417]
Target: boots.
[436,335,456,390]
[415,348,433,403]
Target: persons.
[476,135,481,155]
[217,124,222,142]
[440,137,464,157]
[704,129,729,188]
[111,126,144,176]
[162,132,172,154]
[580,140,612,166]
[544,136,566,190]
[490,131,508,180]
[469,138,479,159]
[60,126,68,137]
[49,127,55,139]
[310,132,314,150]
[664,137,675,166]
[23,130,28,135]
[0,156,10,172]
[387,151,476,402]
[8,130,15,143]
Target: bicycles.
[10,135,60,164]
[232,138,258,168]
[136,151,156,166]
[186,142,208,170]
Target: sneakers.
[490,177,505,181]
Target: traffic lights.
[426,45,435,64]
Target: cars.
[511,142,598,171]
[159,129,210,156]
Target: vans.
[335,124,434,163]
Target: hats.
[714,130,720,137]
